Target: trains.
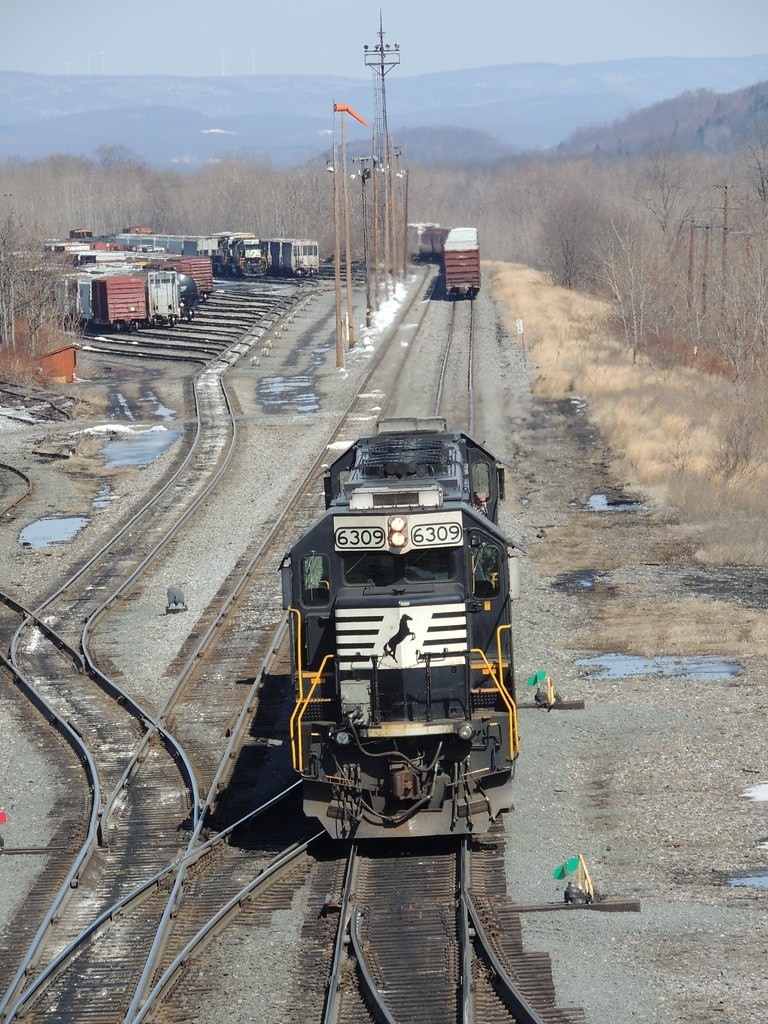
[7,225,321,334]
[406,222,482,300]
[278,416,521,842]
